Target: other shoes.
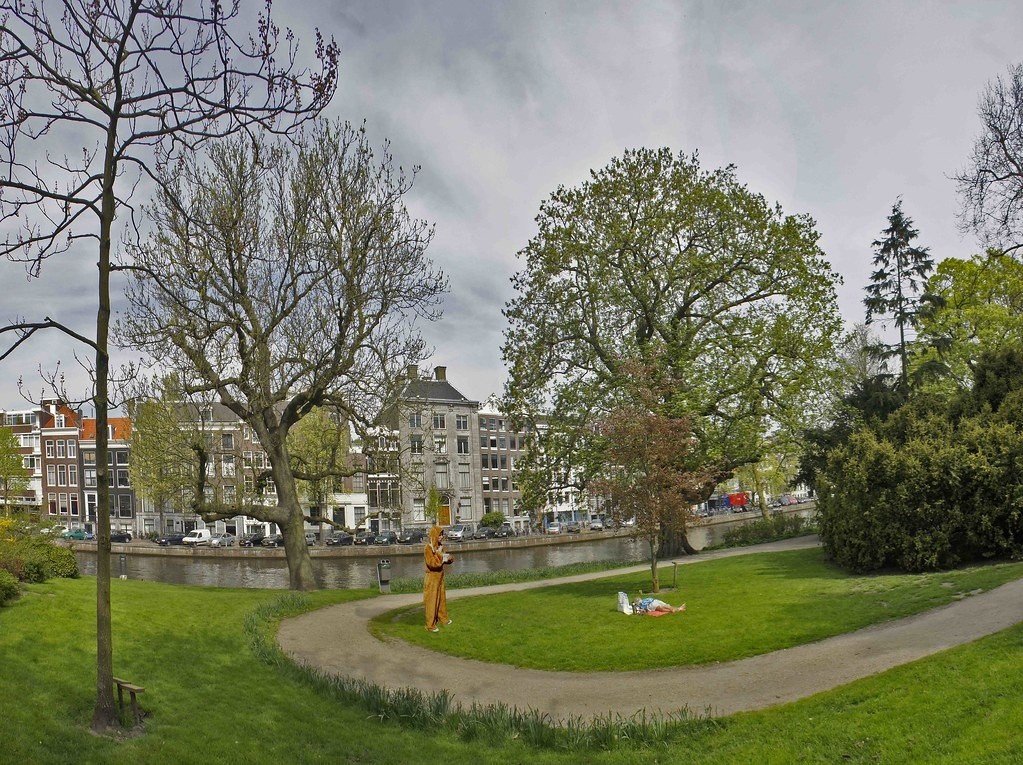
[447,620,452,625]
[432,628,439,632]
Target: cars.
[44,525,70,538]
[154,519,615,547]
[108,531,132,543]
[64,528,95,540]
[691,494,816,518]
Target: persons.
[424,527,454,632]
[634,598,687,613]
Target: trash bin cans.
[377,559,391,593]
